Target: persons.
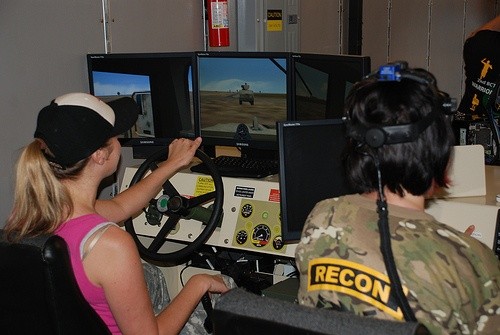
[4,92,238,335]
[451,15,500,118]
[294,60,500,335]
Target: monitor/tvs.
[195,51,294,150]
[294,52,373,119]
[87,52,198,152]
[276,120,363,244]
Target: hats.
[34,92,139,169]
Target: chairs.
[0,229,113,335]
[212,287,431,335]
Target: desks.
[441,165,500,209]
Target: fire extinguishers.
[208,0,230,47]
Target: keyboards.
[188,156,282,179]
